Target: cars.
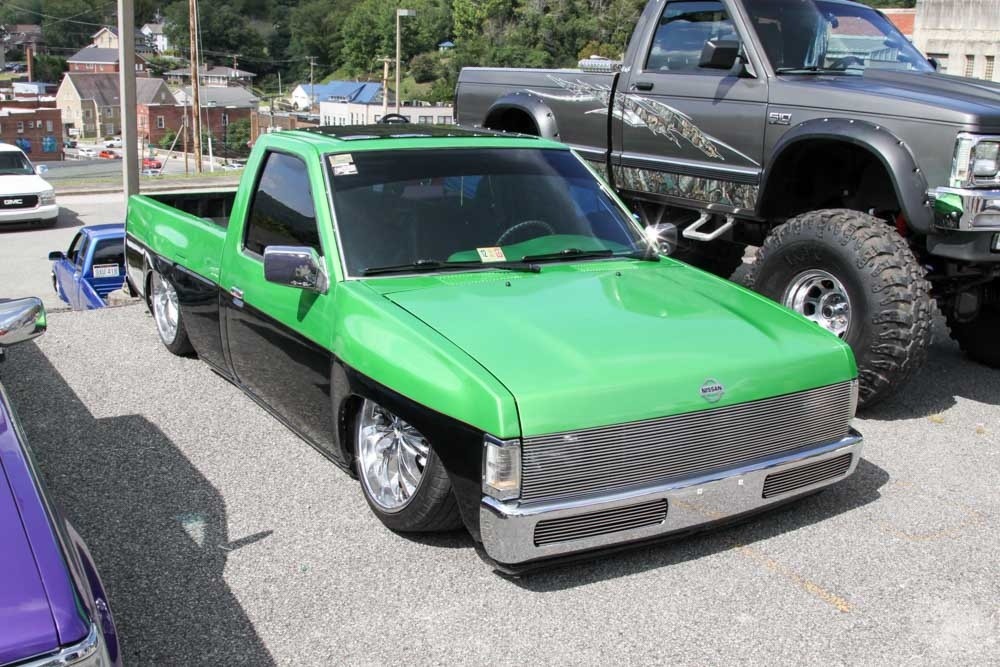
[105,138,123,149]
[0,140,61,229]
[63,137,77,148]
[78,147,96,158]
[141,157,162,170]
[46,222,133,313]
[0,295,131,667]
[100,150,120,160]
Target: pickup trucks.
[446,0,1000,416]
[124,116,866,568]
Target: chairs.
[650,22,704,68]
[410,174,528,261]
[757,22,784,68]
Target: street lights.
[393,8,417,122]
